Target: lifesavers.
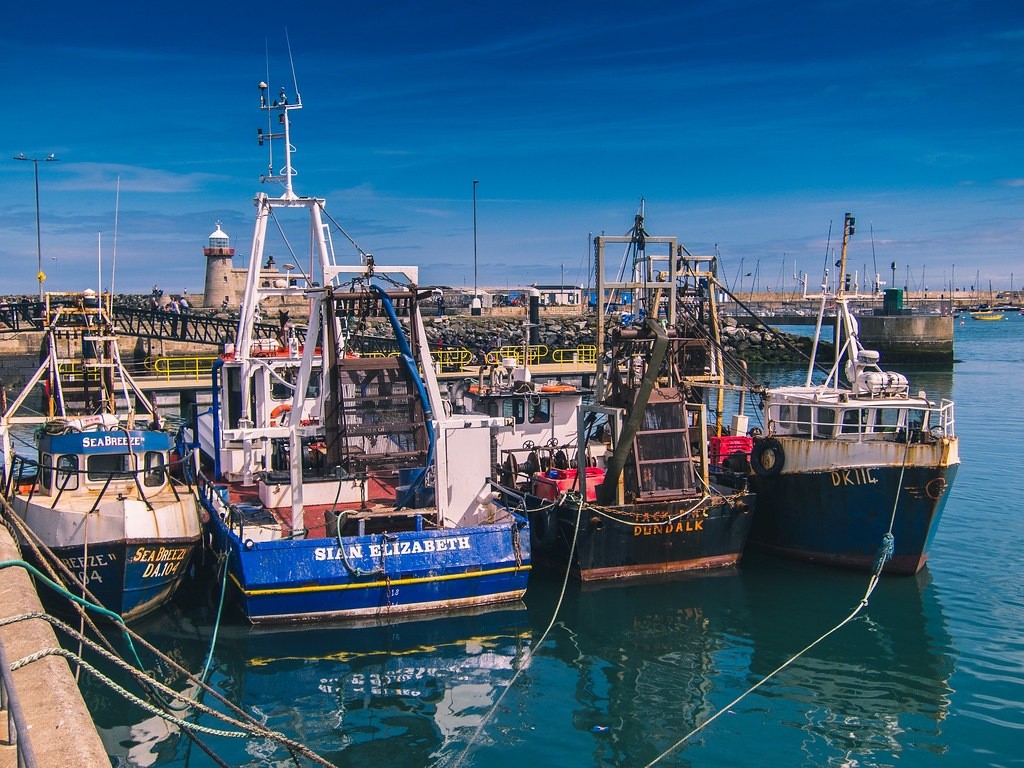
[751,437,785,477]
[530,504,559,552]
[541,384,576,393]
[272,405,292,427]
[206,575,235,614]
[467,385,490,394]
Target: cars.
[996,294,1003,298]
[736,310,753,315]
[499,300,515,306]
[620,310,635,317]
[312,281,320,287]
[798,309,812,316]
[610,311,619,315]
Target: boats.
[930,308,952,314]
[1,311,209,626]
[414,316,758,593]
[992,301,1020,311]
[969,308,994,315]
[971,315,1004,320]
[169,81,533,633]
[597,213,961,577]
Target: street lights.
[13,152,60,302]
[52,257,58,300]
[281,264,295,288]
[587,232,592,303]
[471,180,482,315]
[561,264,566,305]
